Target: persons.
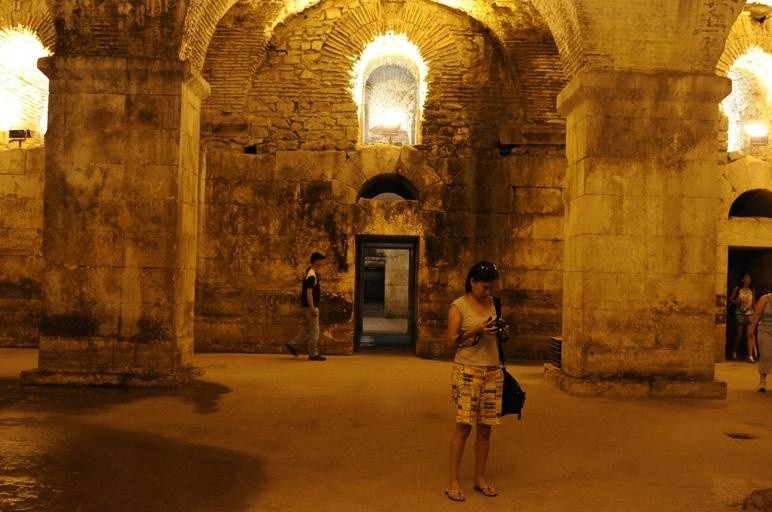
[746,292,772,391]
[442,259,509,502]
[282,253,328,361]
[728,273,758,362]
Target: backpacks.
[502,368,526,416]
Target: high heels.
[733,353,736,359]
[748,356,755,363]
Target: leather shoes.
[309,355,326,361]
[285,342,298,357]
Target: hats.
[310,252,326,263]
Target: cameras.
[493,318,509,343]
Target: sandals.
[446,488,464,501]
[474,484,498,496]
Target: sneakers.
[759,379,767,389]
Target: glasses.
[477,264,498,272]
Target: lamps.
[8,129,35,147]
[749,136,768,158]
[382,123,401,145]
